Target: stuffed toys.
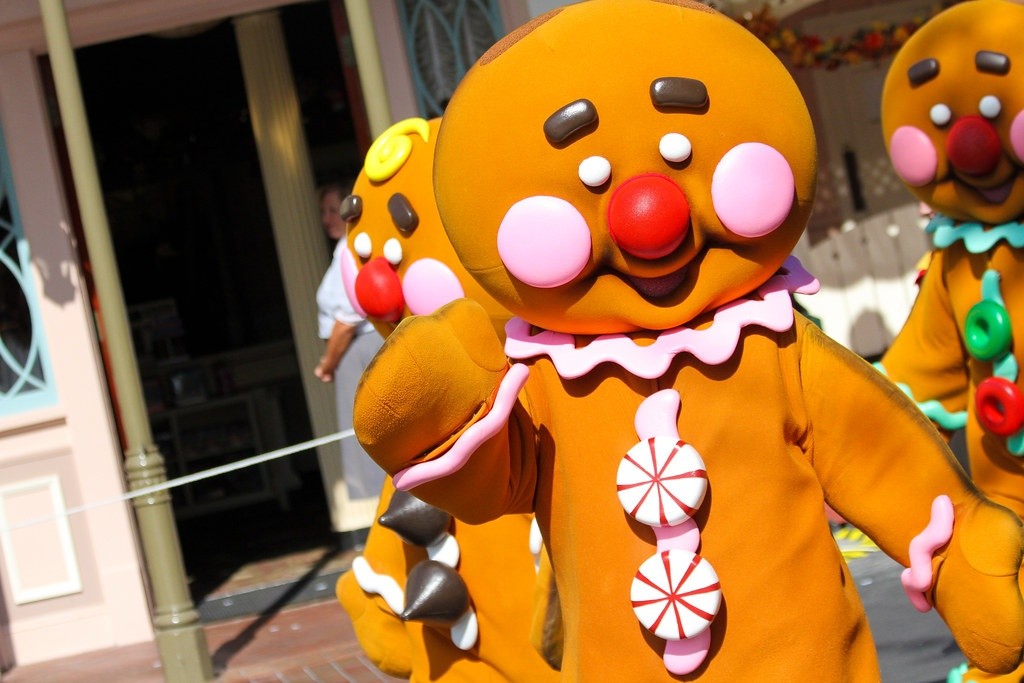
[336,115,562,683]
[872,0,1024,683]
[435,0,1023,683]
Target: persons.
[310,181,391,497]
